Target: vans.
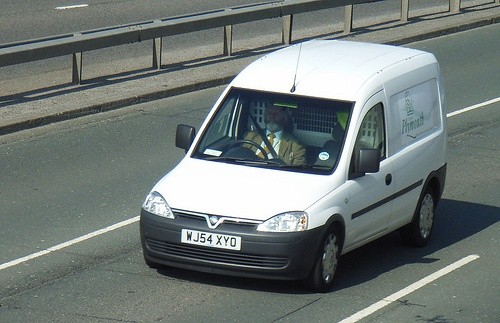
[140,38,448,292]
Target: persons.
[240,102,307,166]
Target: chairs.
[313,122,360,172]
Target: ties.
[257,131,275,158]
